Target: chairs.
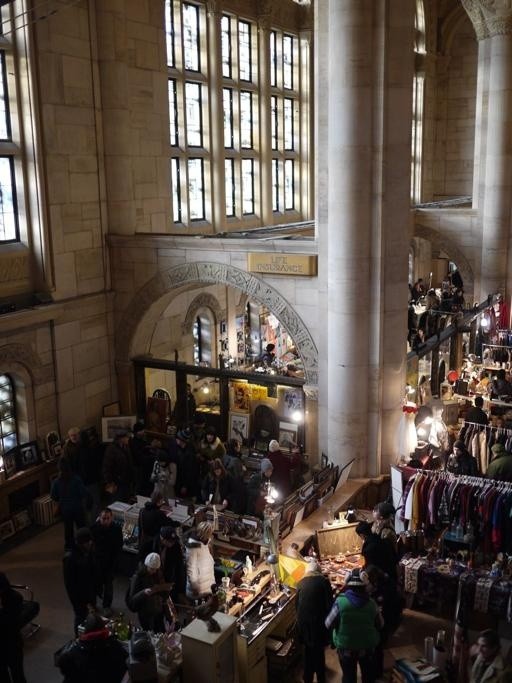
[1,573,42,638]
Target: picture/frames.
[227,410,251,440]
[99,414,138,444]
[103,400,122,416]
[0,430,63,538]
[281,449,357,540]
[277,415,299,446]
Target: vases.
[222,576,231,587]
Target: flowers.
[215,554,237,577]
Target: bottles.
[116,612,129,641]
[426,536,442,568]
[246,555,252,574]
[452,516,474,539]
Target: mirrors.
[253,404,279,444]
[153,387,173,423]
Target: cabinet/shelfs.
[234,583,300,682]
[180,610,240,682]
[266,632,296,681]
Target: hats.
[260,457,273,472]
[160,525,179,540]
[305,561,322,573]
[176,430,192,442]
[269,439,280,453]
[76,527,95,543]
[347,575,366,590]
[144,551,160,568]
[379,502,396,515]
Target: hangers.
[411,329,512,494]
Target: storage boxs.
[314,522,366,586]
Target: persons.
[0,571,28,683]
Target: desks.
[399,549,512,639]
[443,527,485,565]
[76,612,185,682]
